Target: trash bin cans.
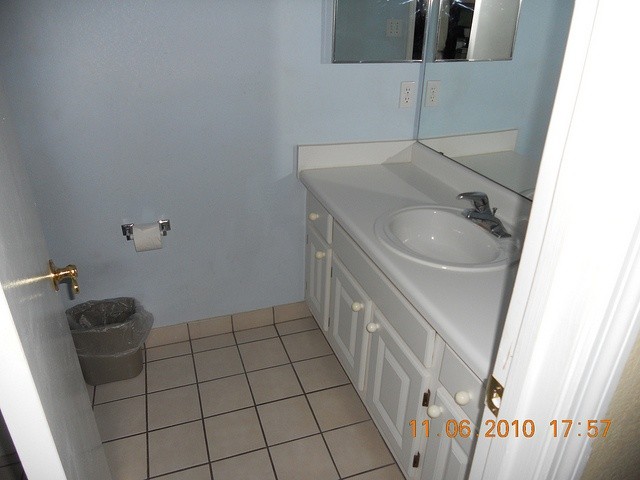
[66,296,153,385]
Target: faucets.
[457,190,507,239]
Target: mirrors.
[330,0,423,63]
[417,0,574,202]
[432,0,522,63]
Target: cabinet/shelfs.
[304,189,333,333]
[409,339,490,480]
[328,218,436,480]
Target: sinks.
[388,209,500,266]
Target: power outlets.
[386,18,402,37]
[425,80,439,107]
[399,81,416,106]
